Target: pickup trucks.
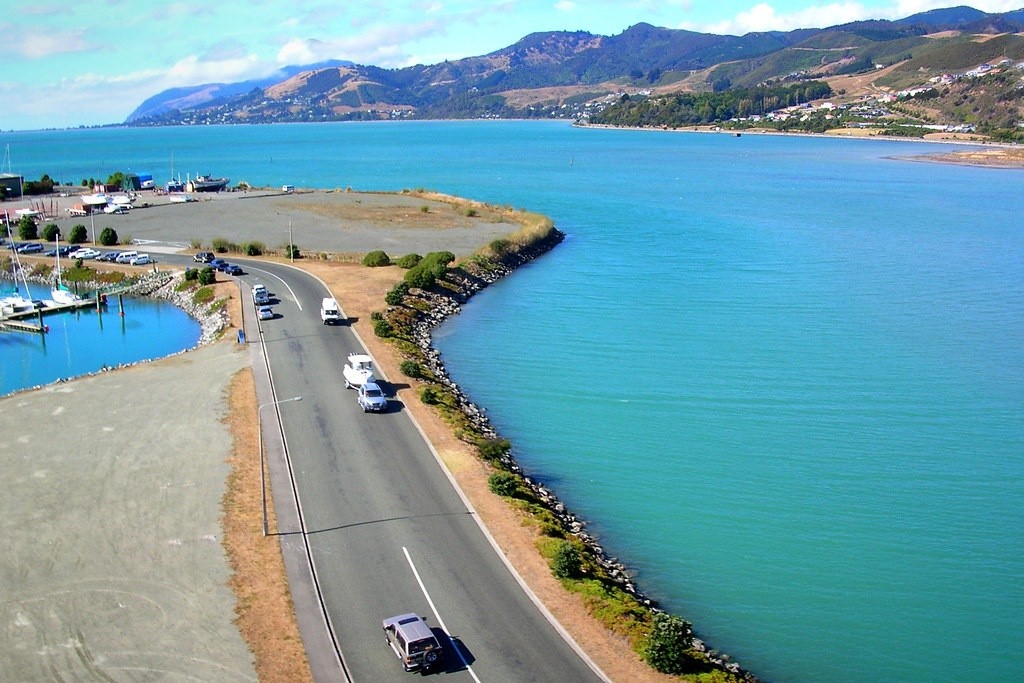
[207,259,230,272]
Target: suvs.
[358,383,388,413]
[193,251,215,264]
[17,243,44,255]
[382,612,442,673]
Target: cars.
[224,265,243,276]
[107,252,122,262]
[44,245,101,260]
[6,242,32,253]
[257,306,274,321]
[252,285,270,305]
[0,237,5,245]
[96,253,108,262]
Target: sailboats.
[51,233,81,304]
[0,209,37,317]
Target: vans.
[115,251,138,265]
[129,254,150,266]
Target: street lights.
[258,397,303,537]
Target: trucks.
[320,297,339,325]
